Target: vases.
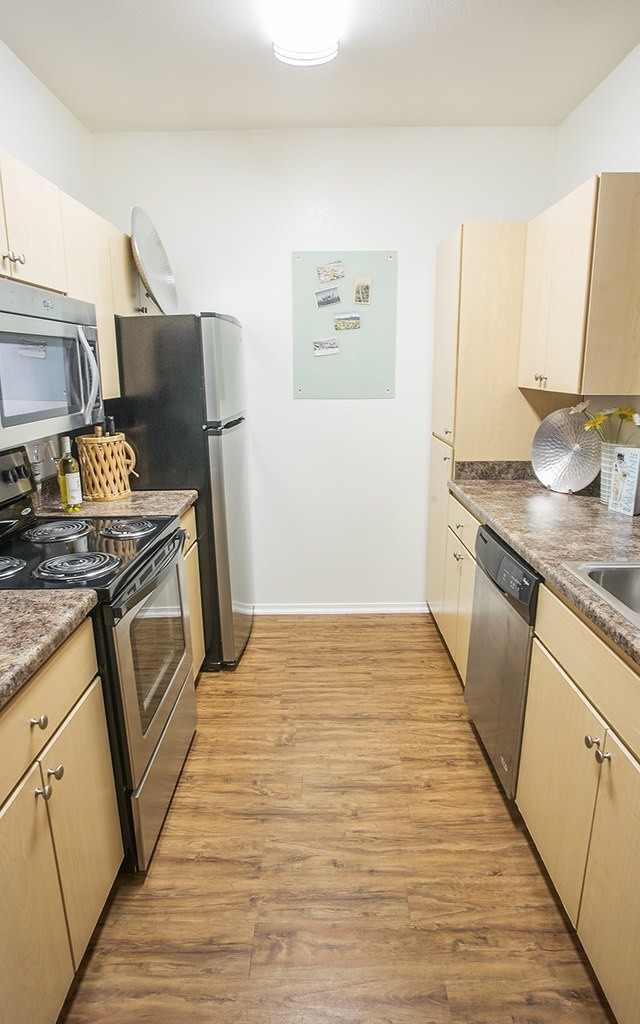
[599,440,638,504]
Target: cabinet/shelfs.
[424,219,560,686]
[515,583,640,1024]
[0,148,161,401]
[0,617,125,1023]
[181,505,206,682]
[517,170,640,395]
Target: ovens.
[85,526,197,874]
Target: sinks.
[562,560,640,631]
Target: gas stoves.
[0,444,179,601]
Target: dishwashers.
[463,525,546,800]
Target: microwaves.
[0,278,105,452]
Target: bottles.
[57,436,84,513]
[105,416,114,437]
[95,426,102,438]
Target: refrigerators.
[113,311,255,673]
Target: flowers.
[569,398,640,445]
